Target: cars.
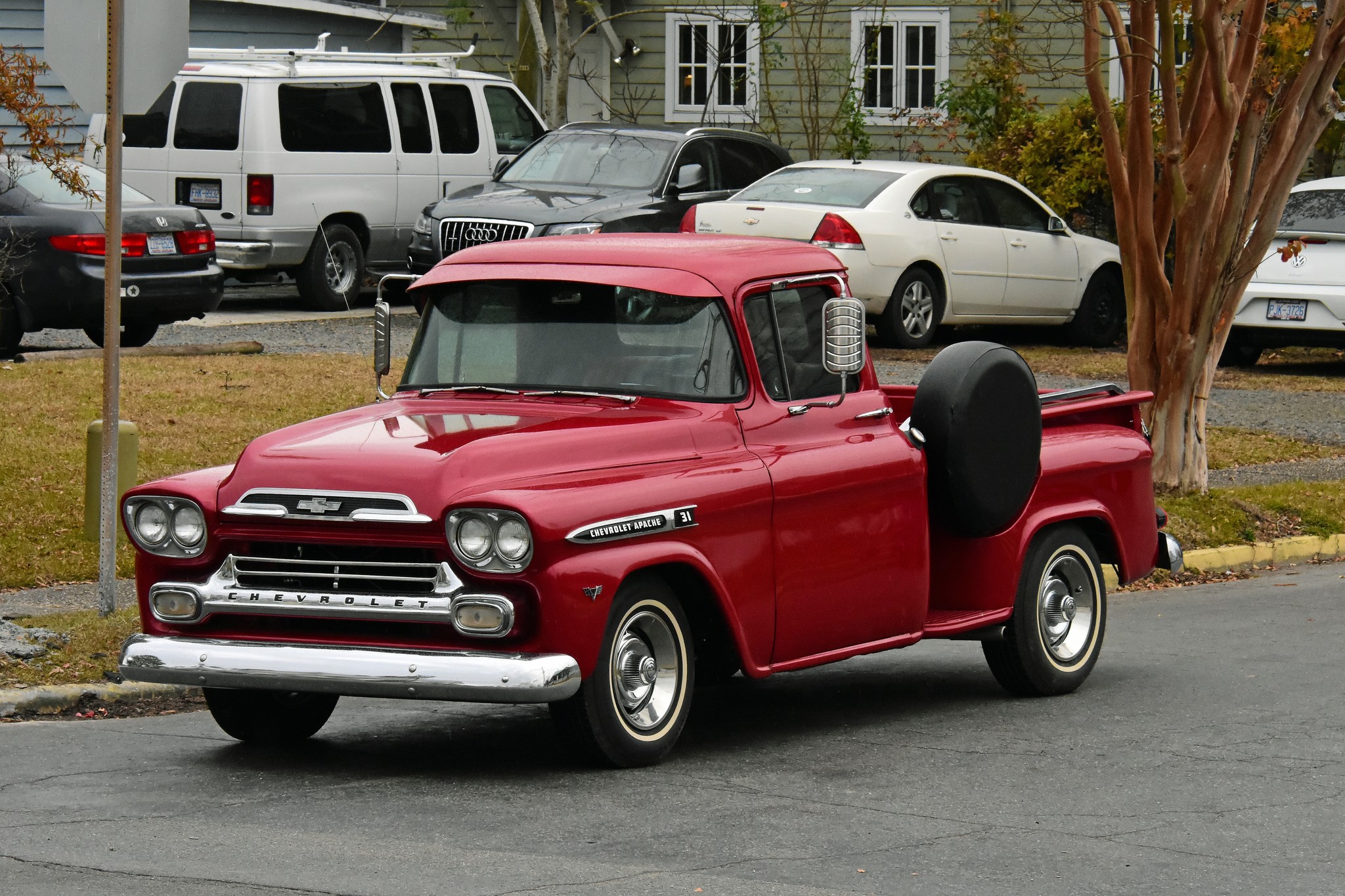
[678,160,1126,349]
[1216,177,1345,370]
[0,149,224,360]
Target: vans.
[83,31,548,313]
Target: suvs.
[404,122,796,316]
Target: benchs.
[546,355,854,409]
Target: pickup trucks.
[115,233,1183,776]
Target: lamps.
[612,51,628,68]
[626,38,641,57]
